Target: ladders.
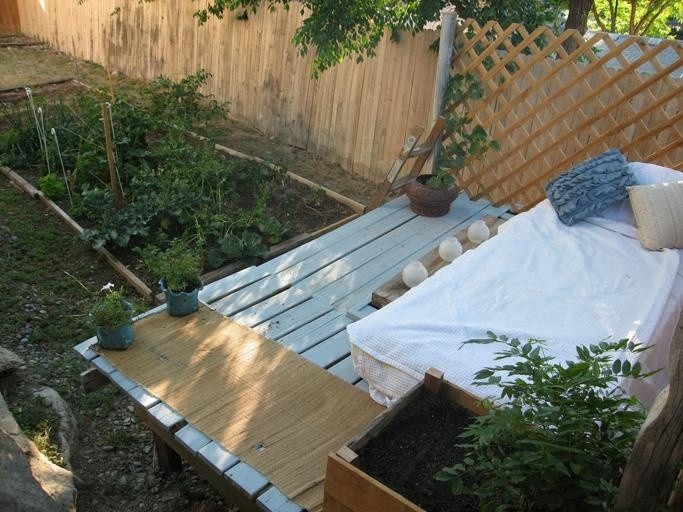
[366,115,446,214]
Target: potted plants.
[405,73,503,217]
[327,334,666,511]
[86,245,206,351]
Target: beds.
[348,160,682,452]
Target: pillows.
[544,147,683,253]
